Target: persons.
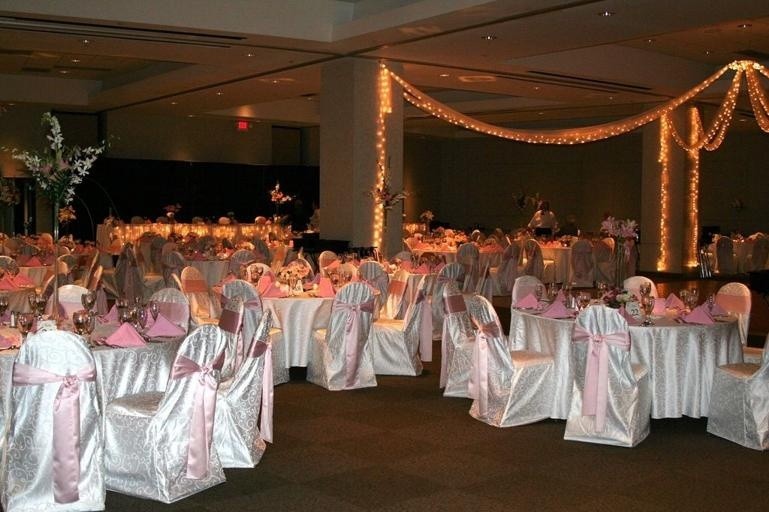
[527,201,556,240]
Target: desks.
[511,297,744,420]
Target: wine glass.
[0,287,161,352]
[232,263,352,294]
[531,278,716,324]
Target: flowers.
[0,111,122,209]
[361,163,415,212]
[268,182,296,206]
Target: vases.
[48,199,66,322]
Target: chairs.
[105,325,228,504]
[296,229,768,396]
[707,335,768,449]
[0,213,296,471]
[563,305,652,448]
[0,330,107,510]
[467,295,558,428]
